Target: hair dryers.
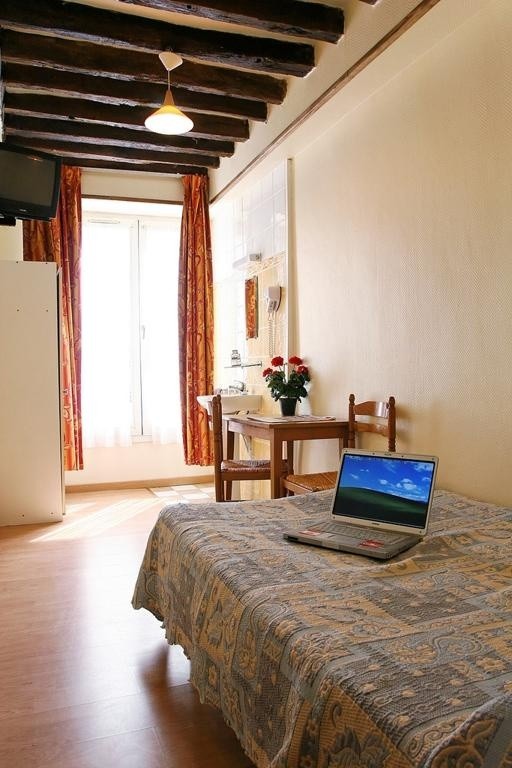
[264,287,281,312]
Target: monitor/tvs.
[0,141,62,221]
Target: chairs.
[280,395,395,495]
[213,394,294,502]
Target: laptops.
[283,447,439,561]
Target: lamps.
[144,51,194,137]
[231,253,260,271]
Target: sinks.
[197,395,261,413]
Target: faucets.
[229,379,246,392]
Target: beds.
[132,487,511,768]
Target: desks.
[224,415,356,499]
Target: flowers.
[262,356,311,403]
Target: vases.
[279,397,297,416]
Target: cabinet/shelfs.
[0,260,65,525]
